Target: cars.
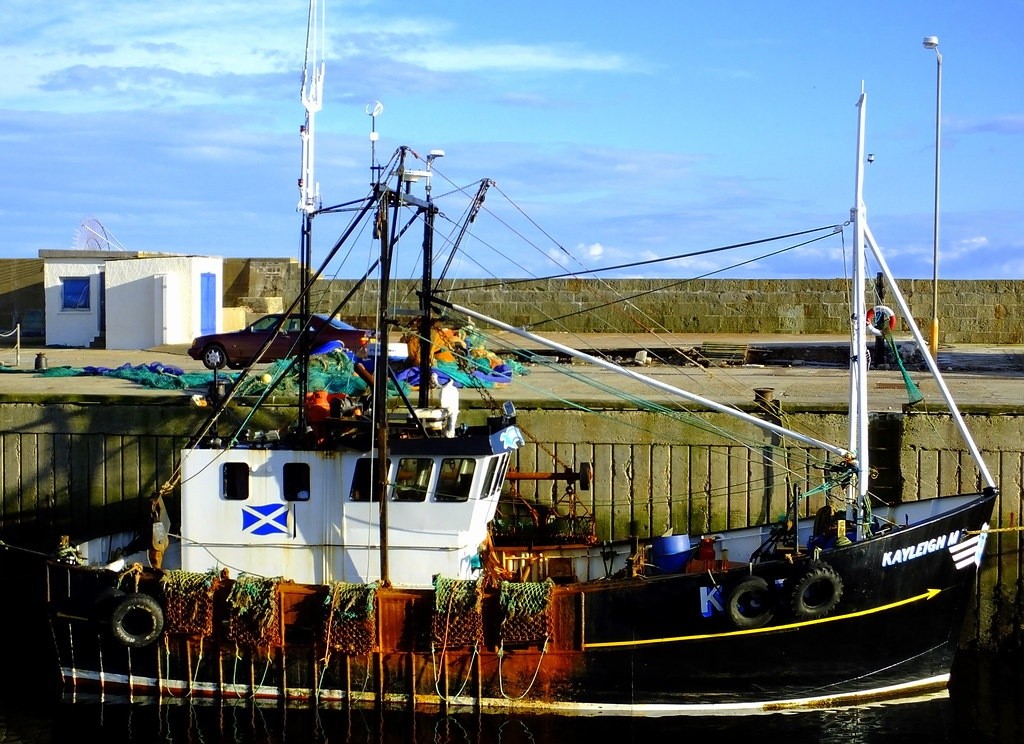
[186,312,369,371]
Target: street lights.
[922,33,943,376]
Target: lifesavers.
[724,576,778,629]
[786,565,847,619]
[868,306,896,337]
[107,593,163,651]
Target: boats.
[46,2,1001,744]
